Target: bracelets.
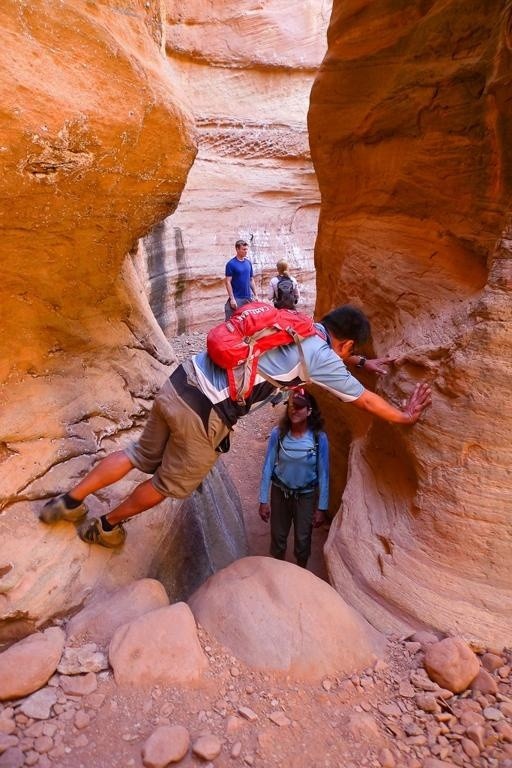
[354,354,367,368]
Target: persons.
[267,257,301,312]
[222,238,259,322]
[35,304,433,551]
[256,390,332,571]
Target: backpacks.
[207,302,327,370]
[274,275,295,308]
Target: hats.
[284,388,312,407]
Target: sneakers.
[79,517,127,549]
[40,494,88,524]
